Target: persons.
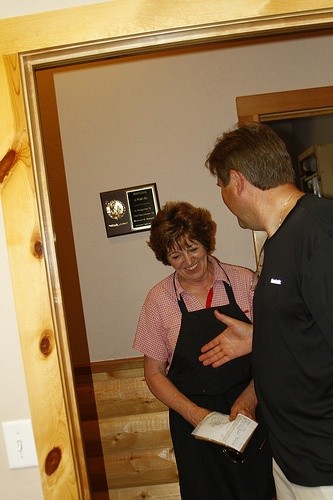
[132,202,277,500]
[198,120,333,500]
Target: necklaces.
[278,189,300,227]
